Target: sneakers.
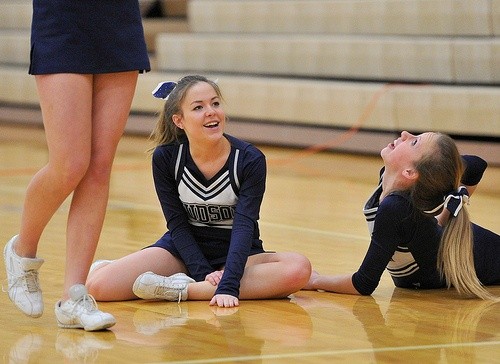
[3,233,45,318]
[54,284,117,332]
[86,259,112,281]
[132,270,196,303]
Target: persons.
[299,130,500,295]
[0,1,151,334]
[84,73,312,307]
